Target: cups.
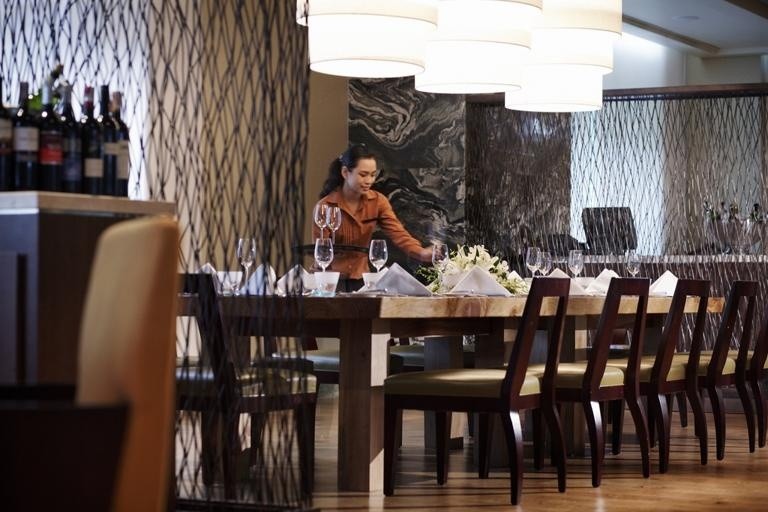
[362,272,378,286]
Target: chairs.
[180,267,767,511]
[0,215,183,511]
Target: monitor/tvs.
[581,206,640,252]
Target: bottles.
[0,82,129,202]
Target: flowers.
[420,245,523,287]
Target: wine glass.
[368,239,389,281]
[313,204,342,298]
[627,254,641,277]
[523,246,593,289]
[238,237,256,296]
[430,243,450,295]
[705,220,764,254]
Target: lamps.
[288,0,621,111]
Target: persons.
[313,145,444,292]
